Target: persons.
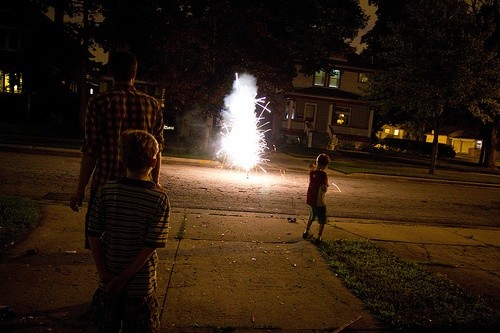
[301,153,332,246]
[69,49,165,249]
[87,128,172,333]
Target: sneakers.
[302,233,313,239]
[314,239,324,246]
